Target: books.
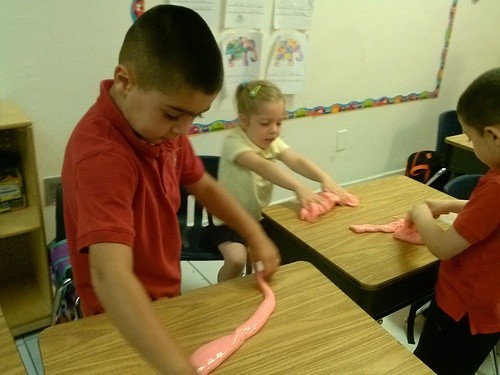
[0,154,27,213]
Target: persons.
[62,5,279,375]
[210,80,353,282]
[406,67,500,375]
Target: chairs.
[176,155,252,276]
[434,110,463,170]
[444,174,484,199]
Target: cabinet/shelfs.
[0,103,54,337]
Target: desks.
[445,133,489,180]
[262,175,460,321]
[37,260,437,375]
